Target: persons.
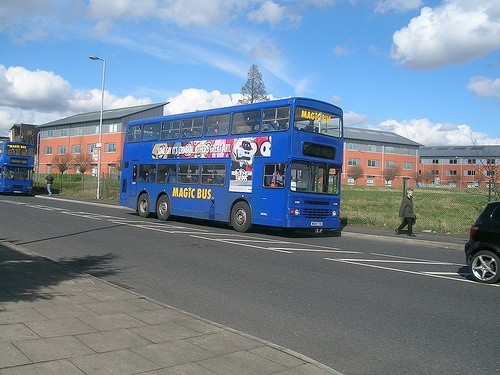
[45,173,54,197]
[395,188,416,236]
[208,197,215,219]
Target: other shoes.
[408,234,416,237]
[396,230,400,235]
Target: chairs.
[139,125,228,141]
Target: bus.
[119,97,345,233]
[0,141,34,195]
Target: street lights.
[88,55,106,200]
[36,131,42,182]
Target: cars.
[464,202,500,284]
